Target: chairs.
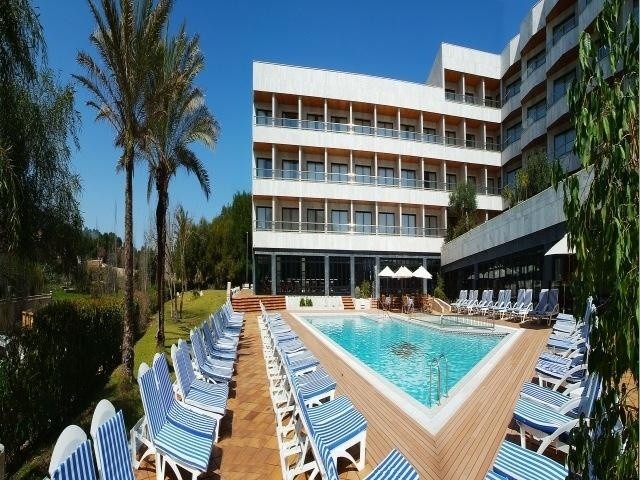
[49,298,245,480]
[256,298,420,480]
[451,289,626,480]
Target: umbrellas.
[413,265,433,295]
[378,266,395,291]
[544,232,590,271]
[396,265,413,295]
